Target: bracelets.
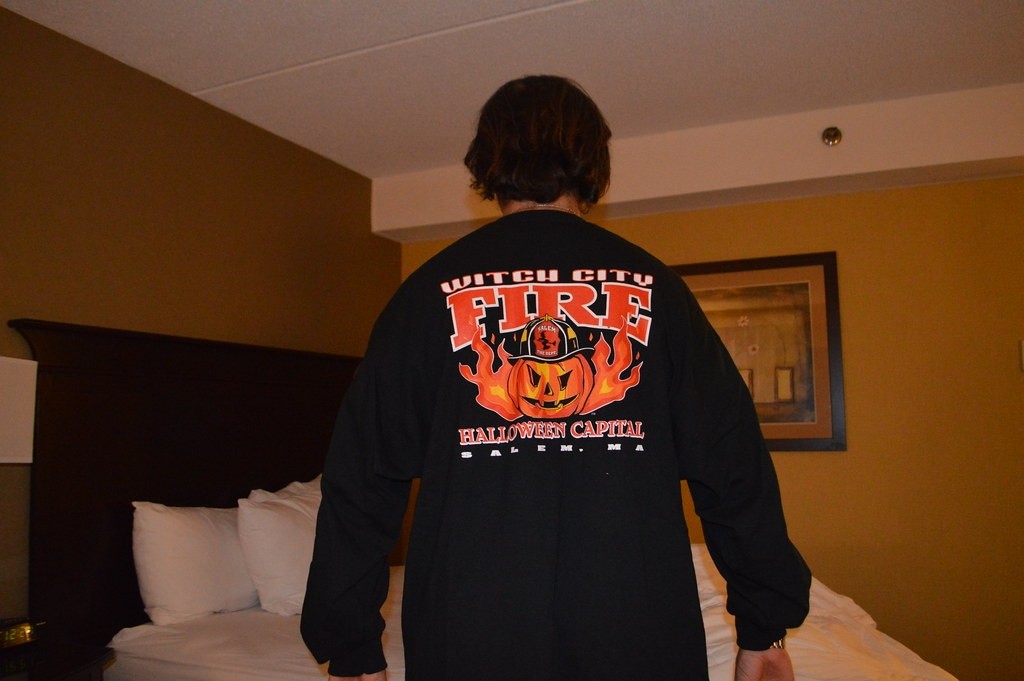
[771,636,786,649]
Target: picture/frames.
[668,250,846,454]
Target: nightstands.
[0,637,114,681]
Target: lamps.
[0,355,48,652]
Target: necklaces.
[516,204,582,218]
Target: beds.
[6,318,962,681]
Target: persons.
[300,74,812,681]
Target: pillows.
[129,472,323,629]
